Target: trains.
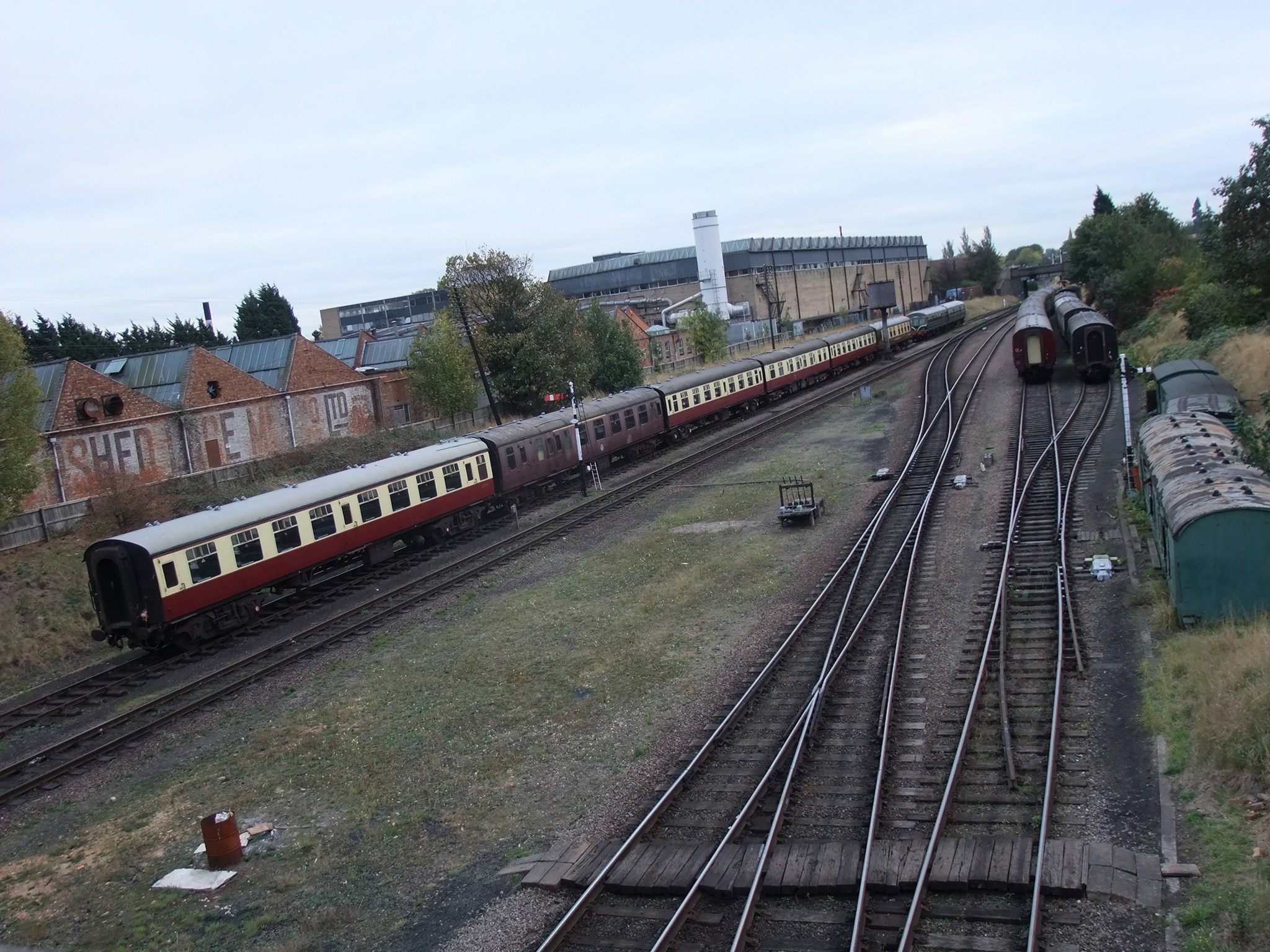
[82,300,967,656]
[1054,283,1120,383]
[1132,352,1270,631]
[1011,281,1069,384]
